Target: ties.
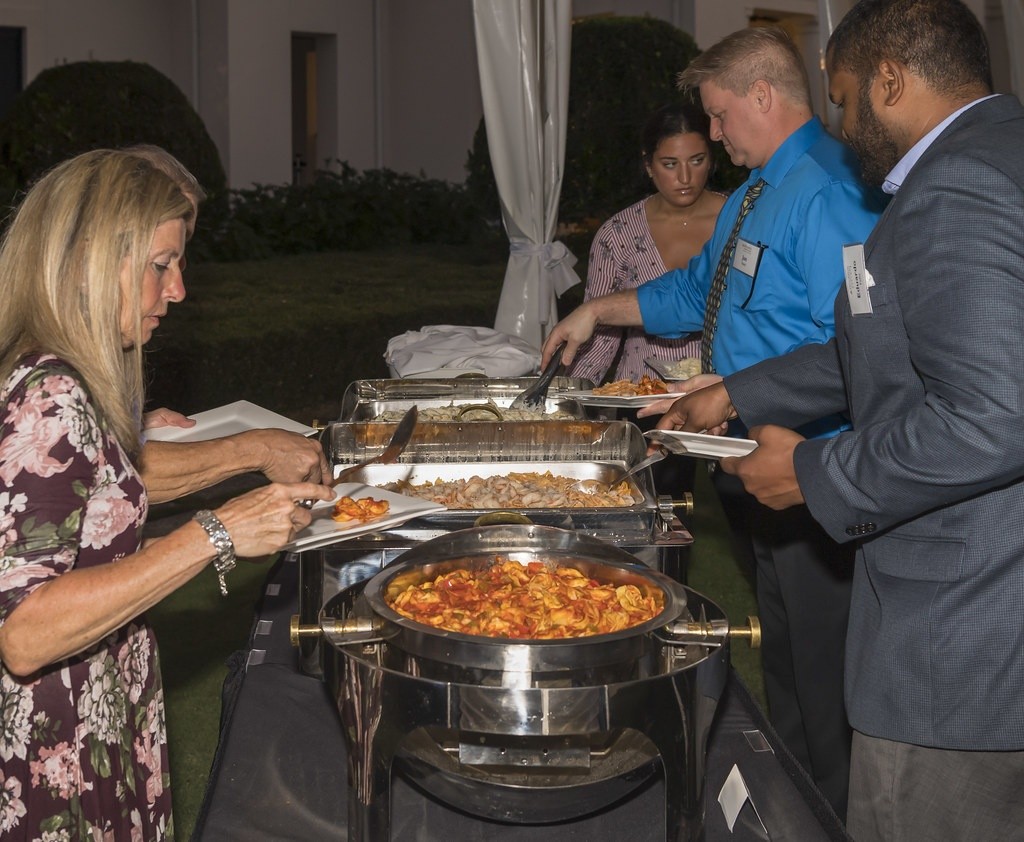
[699,178,764,378]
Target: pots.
[364,548,687,672]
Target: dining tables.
[188,552,855,842]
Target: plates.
[642,429,759,460]
[642,358,692,380]
[558,390,685,408]
[141,400,318,442]
[275,481,448,555]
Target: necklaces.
[683,222,687,226]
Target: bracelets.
[193,509,237,596]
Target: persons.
[646,2,1024,842]
[542,27,882,828]
[126,143,335,504]
[0,152,337,842]
[562,111,753,592]
